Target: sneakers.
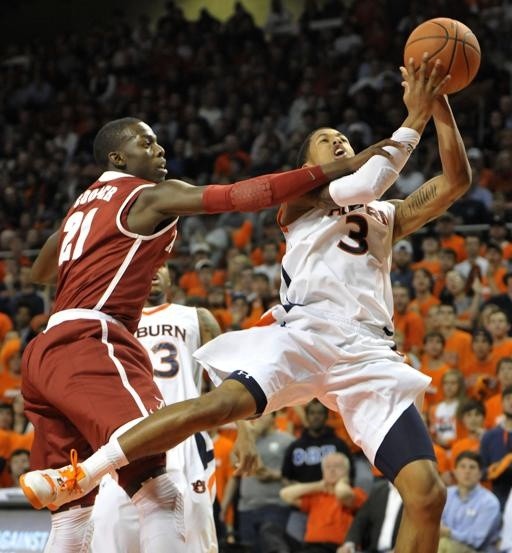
[19,449,93,511]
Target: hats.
[394,240,412,254]
[191,243,214,270]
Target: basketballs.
[404,17,481,94]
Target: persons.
[1,2,512,552]
